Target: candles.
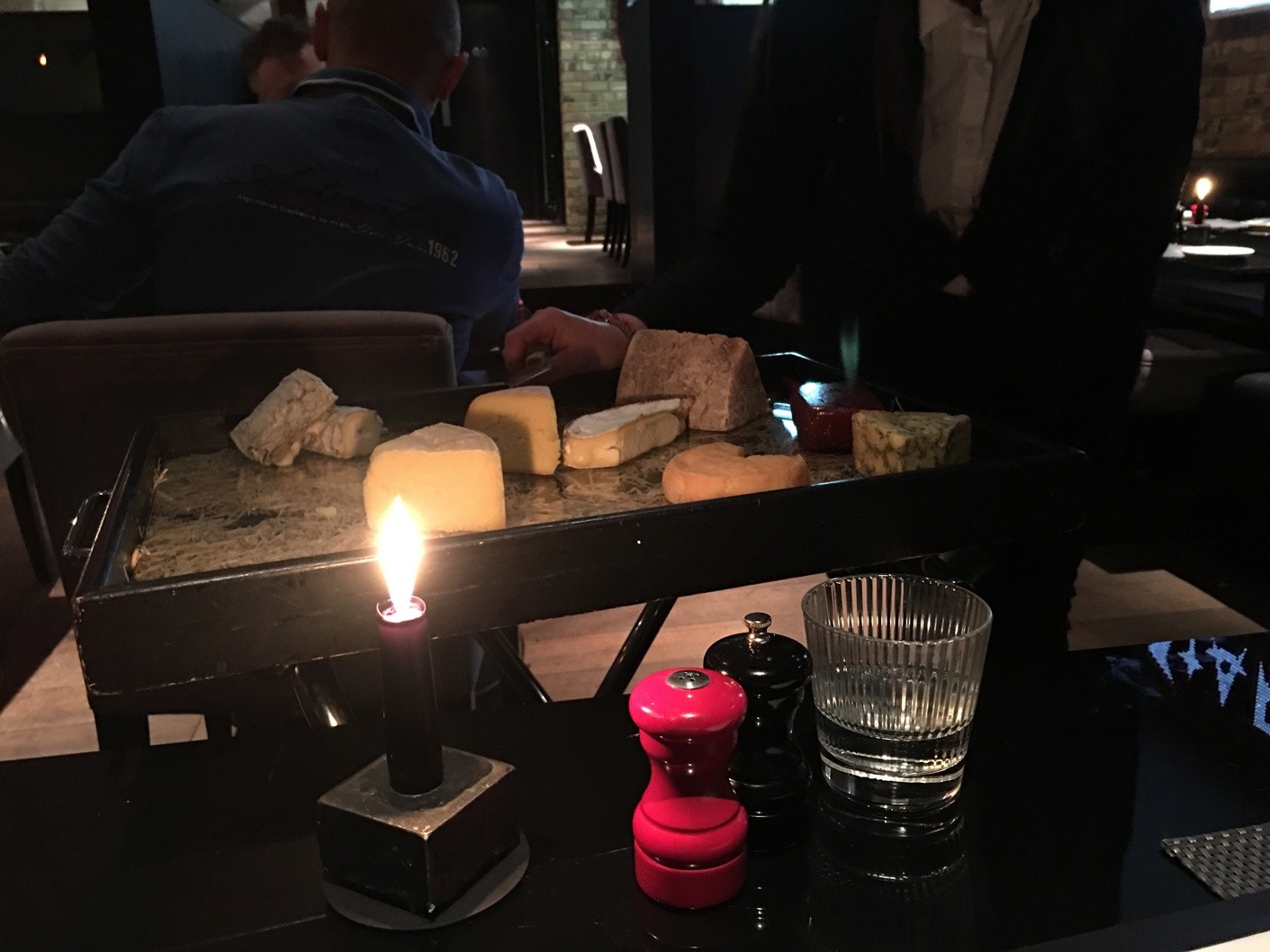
[1193,177,1214,224]
[372,490,443,798]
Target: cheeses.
[361,421,505,532]
[463,385,560,475]
[792,381,972,476]
[229,369,386,468]
[615,328,772,432]
[661,440,811,503]
[562,397,694,470]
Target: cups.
[802,572,993,811]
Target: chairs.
[577,116,630,268]
[0,311,485,746]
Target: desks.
[0,632,1270,952]
[1152,218,1270,316]
[61,348,1089,760]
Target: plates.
[1182,245,1255,257]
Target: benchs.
[1137,154,1270,439]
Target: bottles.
[703,612,813,851]
[629,668,750,908]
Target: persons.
[0,0,525,386]
[502,0,1203,452]
[244,13,324,104]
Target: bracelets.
[590,309,635,338]
